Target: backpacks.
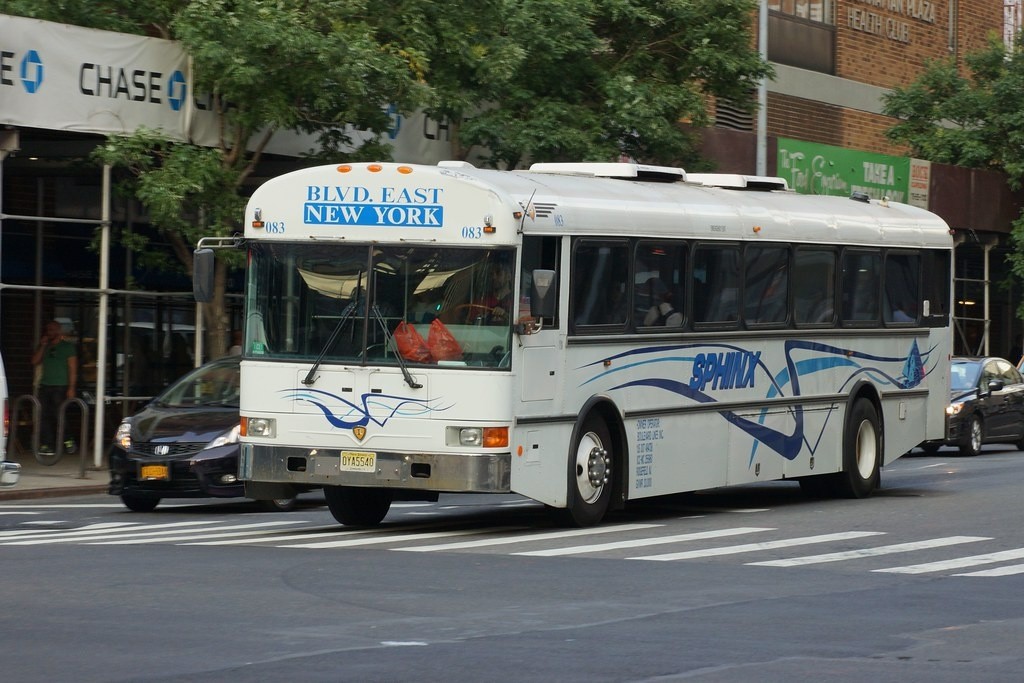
[651,306,680,327]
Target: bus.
[191,159,955,530]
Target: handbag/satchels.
[393,321,431,364]
[427,319,462,364]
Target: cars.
[1011,355,1024,376]
[907,356,1024,456]
[108,355,328,511]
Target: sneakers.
[64,438,76,454]
[40,445,55,452]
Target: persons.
[30,321,78,456]
[643,288,683,327]
[471,261,513,322]
